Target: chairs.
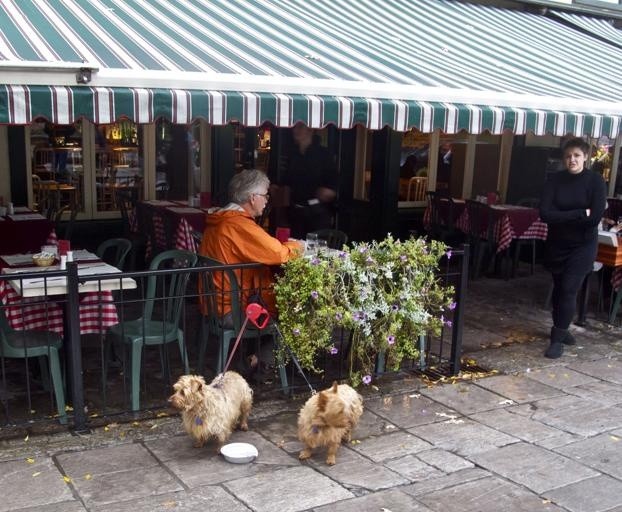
[95,237,134,270]
[407,177,428,201]
[194,253,288,396]
[1,300,73,424]
[428,191,461,251]
[30,166,145,220]
[465,199,505,279]
[310,228,348,259]
[479,189,503,205]
[114,249,199,413]
[1,168,272,254]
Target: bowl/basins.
[220,442,259,464]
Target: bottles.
[60,255,67,271]
[66,251,73,262]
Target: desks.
[440,198,547,278]
[544,224,622,329]
[7,248,137,425]
[274,233,354,272]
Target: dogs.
[166,370,255,455]
[296,380,365,466]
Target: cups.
[303,232,329,256]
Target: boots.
[543,324,577,360]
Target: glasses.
[251,193,270,200]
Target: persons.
[436,139,453,182]
[539,138,607,358]
[197,168,305,389]
[267,123,342,240]
[399,153,418,181]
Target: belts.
[290,198,320,208]
